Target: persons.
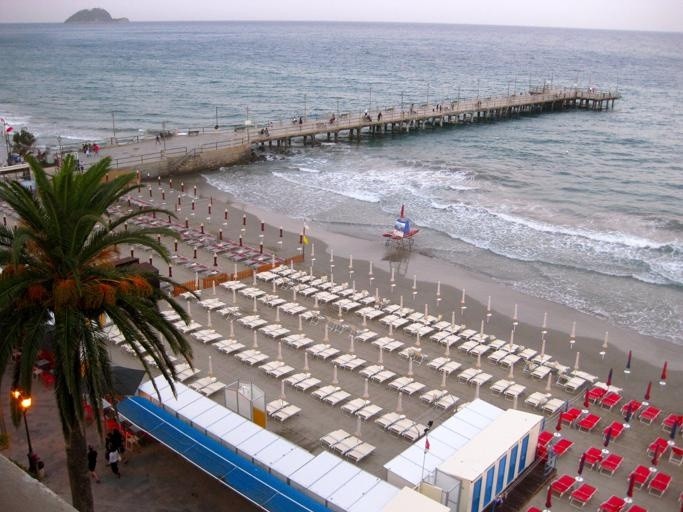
[8,143,100,171]
[433,104,441,111]
[364,109,383,122]
[259,121,273,136]
[156,136,161,144]
[87,446,101,484]
[105,428,123,478]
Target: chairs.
[520,349,682,510]
[102,265,524,464]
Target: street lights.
[11,385,37,480]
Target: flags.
[425,438,429,449]
